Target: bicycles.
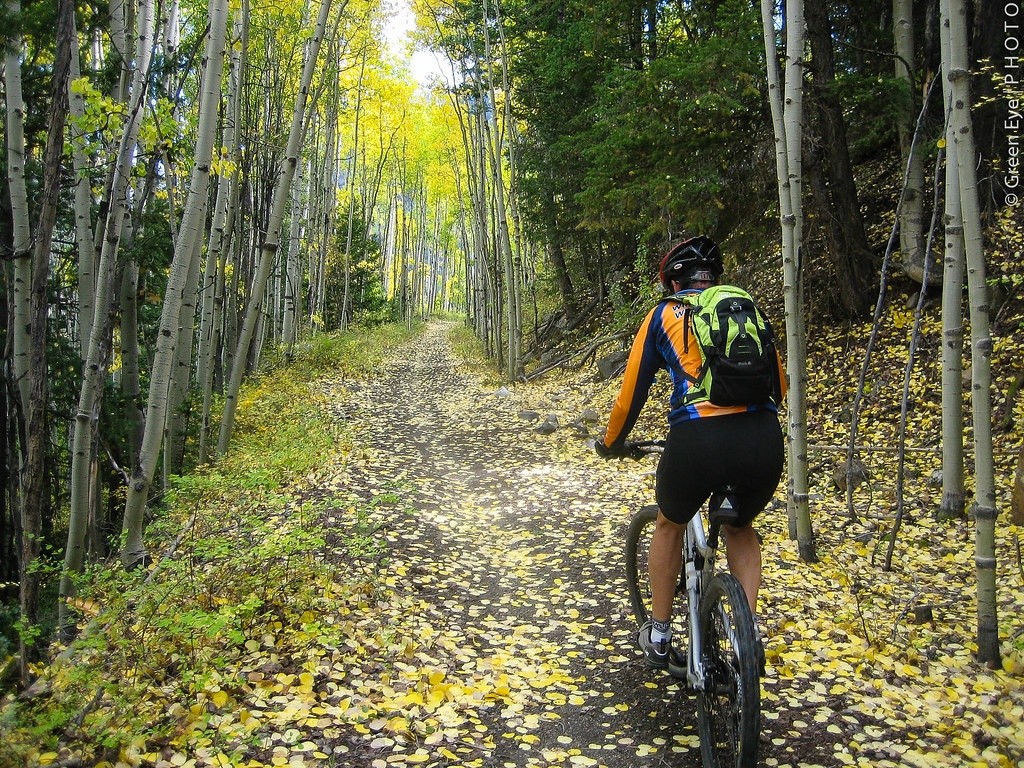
[597,438,767,768]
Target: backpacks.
[658,285,776,408]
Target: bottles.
[686,558,697,591]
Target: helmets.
[659,233,725,287]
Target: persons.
[598,232,788,681]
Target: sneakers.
[637,620,673,670]
[732,636,768,677]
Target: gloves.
[594,439,624,461]
